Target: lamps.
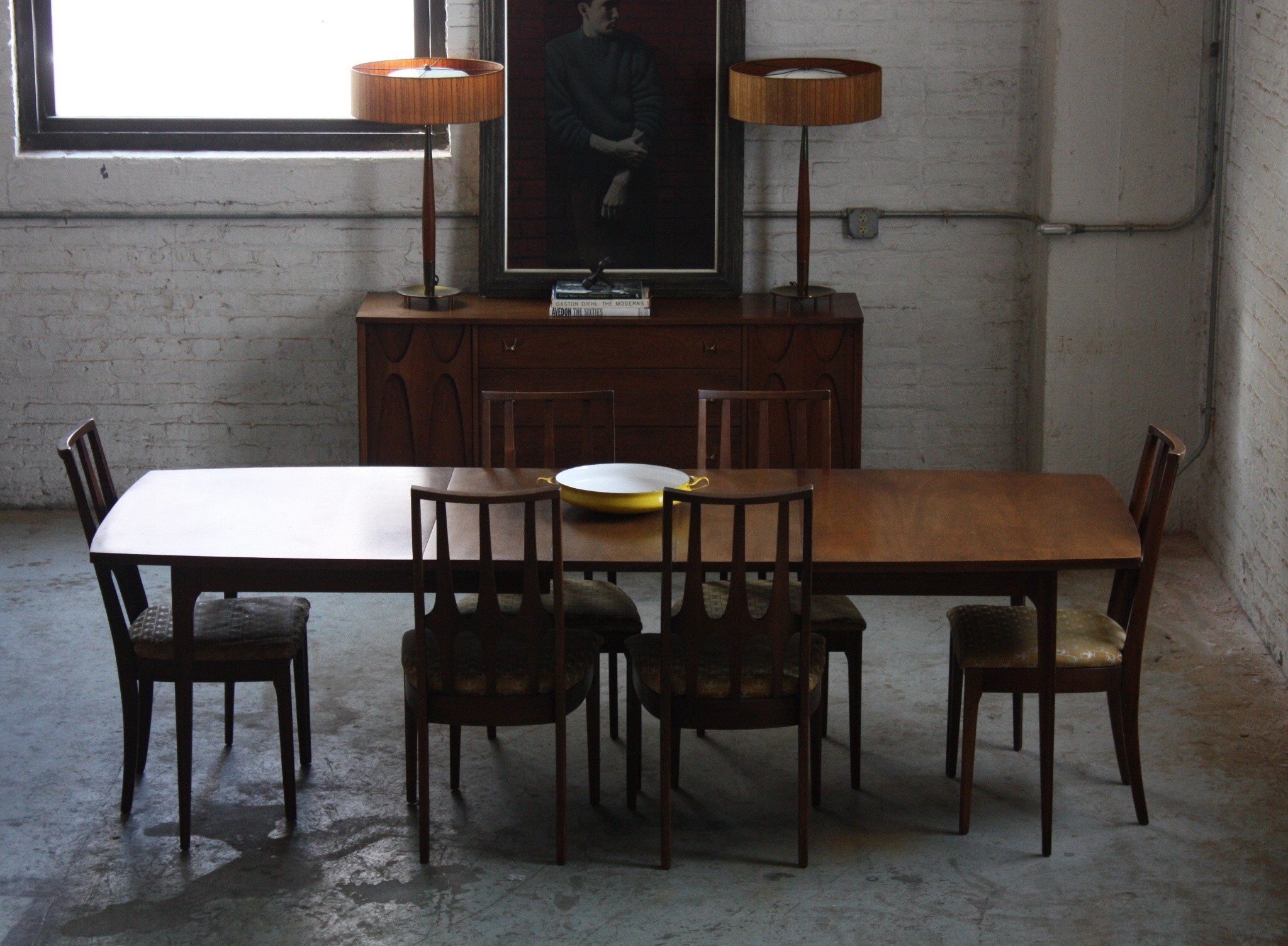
[728,57,883,318]
[352,55,507,313]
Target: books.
[549,279,651,317]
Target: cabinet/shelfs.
[354,291,864,472]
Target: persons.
[537,0,668,268]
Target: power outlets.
[846,208,882,241]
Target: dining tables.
[90,468,1150,855]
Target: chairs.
[56,414,313,833]
[402,385,865,867]
[944,419,1185,840]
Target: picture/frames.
[480,0,746,300]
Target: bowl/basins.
[535,464,709,513]
